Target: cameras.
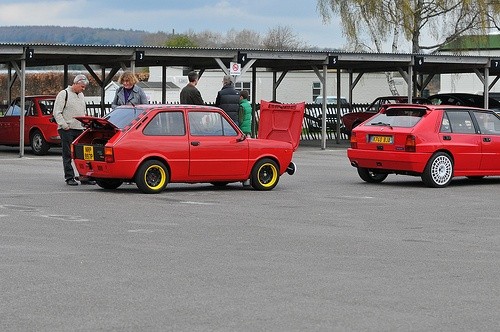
[49,114,57,124]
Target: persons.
[111,71,147,108]
[239,90,252,137]
[52,74,97,185]
[216,75,240,126]
[179,71,204,105]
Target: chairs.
[33,103,47,115]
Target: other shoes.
[65,178,78,185]
[80,179,96,185]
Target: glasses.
[222,81,223,83]
